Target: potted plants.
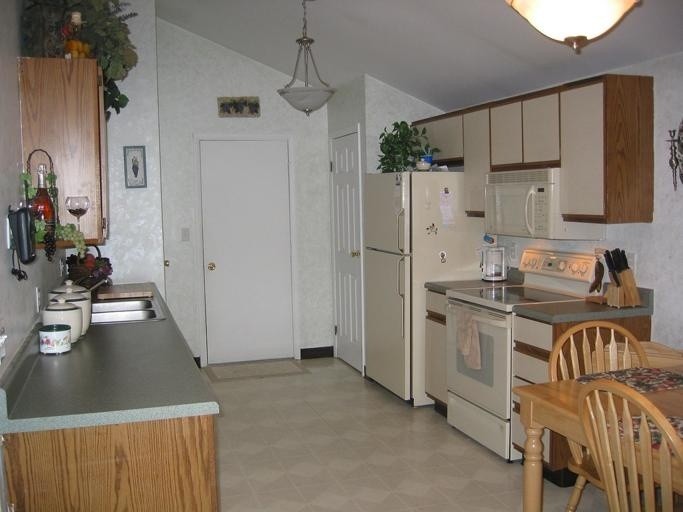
[413,141,442,172]
[378,117,440,171]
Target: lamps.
[275,0,338,119]
[508,0,641,52]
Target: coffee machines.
[482,244,508,281]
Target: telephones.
[8,180,36,265]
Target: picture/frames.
[122,145,148,190]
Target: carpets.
[202,357,313,384]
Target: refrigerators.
[360,174,481,403]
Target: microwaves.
[485,168,605,241]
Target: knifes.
[603,247,630,286]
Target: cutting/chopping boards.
[97,284,153,299]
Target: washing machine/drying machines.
[447,249,601,461]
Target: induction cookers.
[445,284,586,312]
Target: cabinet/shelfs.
[558,76,654,223]
[409,113,463,160]
[460,107,489,217]
[419,285,446,418]
[15,54,111,251]
[487,90,560,168]
[510,313,653,488]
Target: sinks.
[86,310,166,325]
[92,296,152,312]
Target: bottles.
[37,278,92,355]
[65,12,88,59]
[31,164,55,240]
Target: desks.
[510,363,682,511]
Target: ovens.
[445,299,521,464]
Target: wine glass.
[64,195,89,237]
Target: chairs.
[546,320,660,512]
[577,377,683,512]
